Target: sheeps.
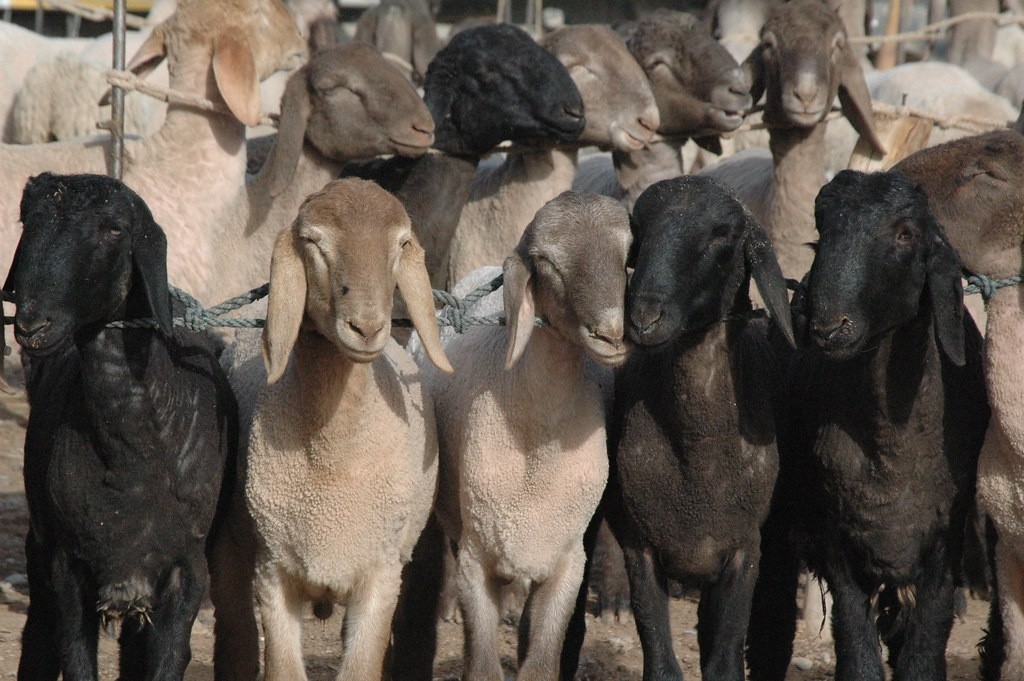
[604,176,799,681]
[406,191,634,680]
[886,126,1023,681]
[0,0,887,623]
[786,169,991,681]
[2,171,260,680]
[220,177,455,681]
